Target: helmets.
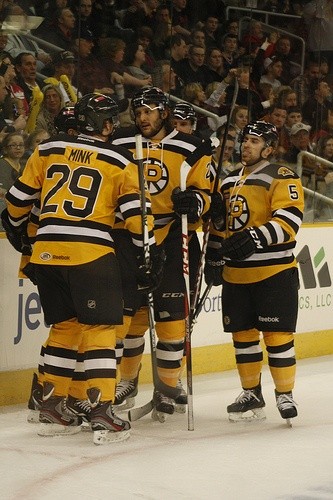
[54,107,79,133]
[73,94,118,133]
[170,102,196,120]
[131,86,169,113]
[243,119,278,147]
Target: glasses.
[5,143,25,149]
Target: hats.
[264,56,284,68]
[51,51,78,64]
[290,122,309,135]
[74,29,97,41]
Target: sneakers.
[274,388,298,427]
[112,363,142,413]
[173,379,187,413]
[26,372,43,423]
[87,387,131,446]
[151,388,174,423]
[227,373,267,422]
[66,394,92,431]
[37,381,82,436]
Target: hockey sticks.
[127,134,165,424]
[127,280,214,423]
[184,70,239,357]
[180,135,221,431]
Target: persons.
[0,0,333,227]
[3,90,224,445]
[203,119,305,428]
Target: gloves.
[0,208,33,256]
[218,230,256,260]
[202,192,223,223]
[170,190,199,214]
[204,263,224,286]
[135,249,165,293]
